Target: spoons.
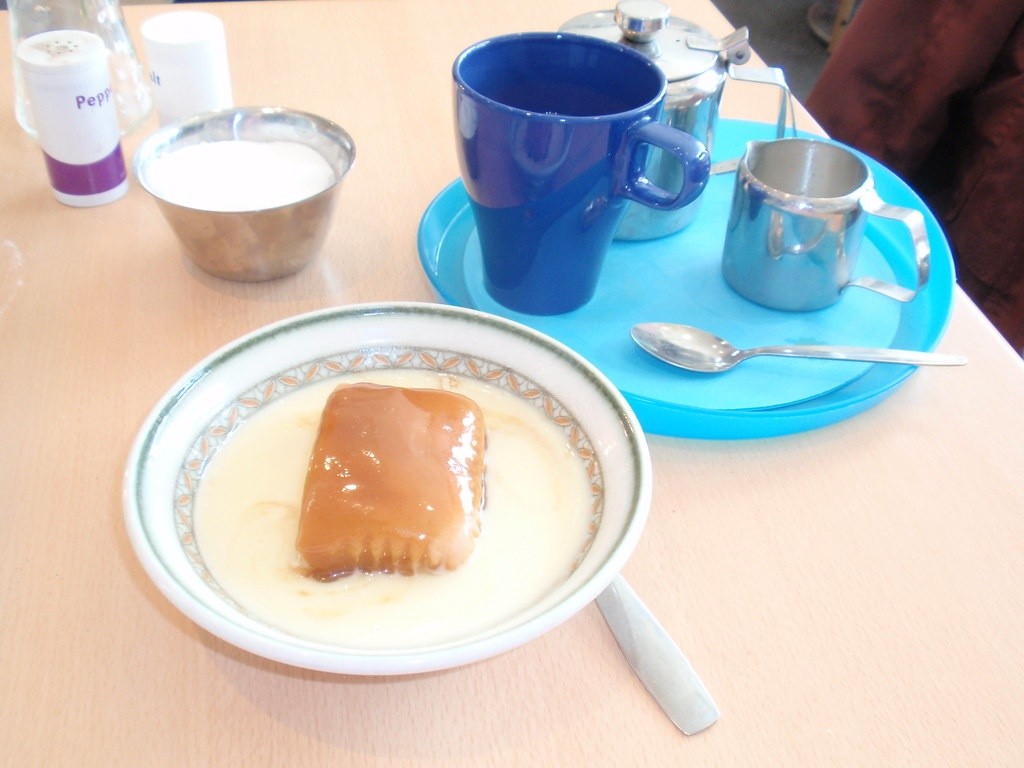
[630,321,968,372]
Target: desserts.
[296,381,488,581]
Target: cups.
[557,0,796,240]
[721,139,930,313]
[453,33,710,316]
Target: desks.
[0,0,1024,768]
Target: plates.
[122,302,651,676]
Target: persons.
[803,0,1024,359]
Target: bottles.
[137,11,234,131]
[5,1,151,140]
[17,31,130,207]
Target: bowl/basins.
[130,106,356,282]
